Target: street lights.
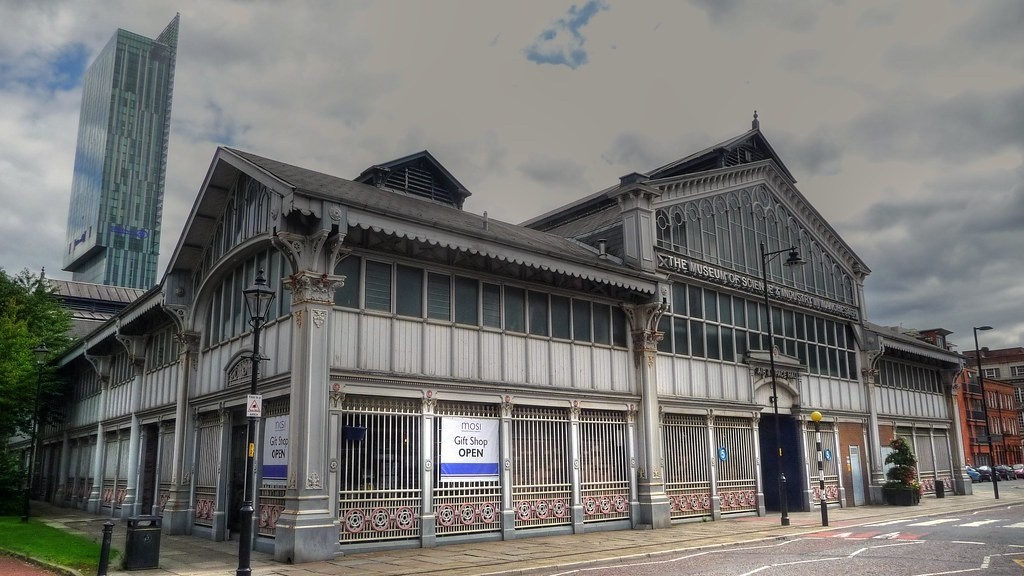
[760,240,807,526]
[236,269,276,576]
[973,326,999,500]
[20,338,51,522]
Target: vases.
[345,425,368,441]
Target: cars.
[1012,464,1024,478]
[979,467,1001,482]
[965,466,982,482]
[997,464,1016,480]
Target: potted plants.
[883,436,923,506]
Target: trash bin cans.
[124,514,163,570]
[935,480,944,498]
